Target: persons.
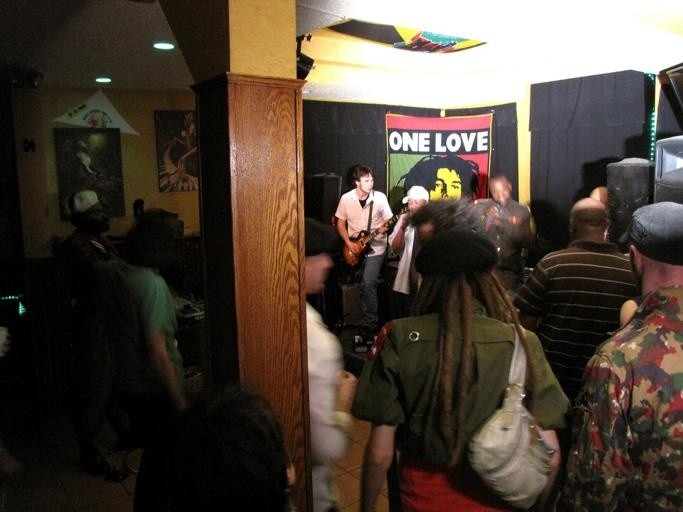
[1,194,205,483]
[306,165,681,510]
[132,388,295,510]
[395,154,481,202]
[73,140,98,181]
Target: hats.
[66,189,101,213]
[305,217,338,258]
[412,228,501,277]
[616,200,683,266]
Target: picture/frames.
[53,125,126,222]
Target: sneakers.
[74,449,129,480]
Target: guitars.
[341,206,409,270]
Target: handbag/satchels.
[466,320,561,509]
